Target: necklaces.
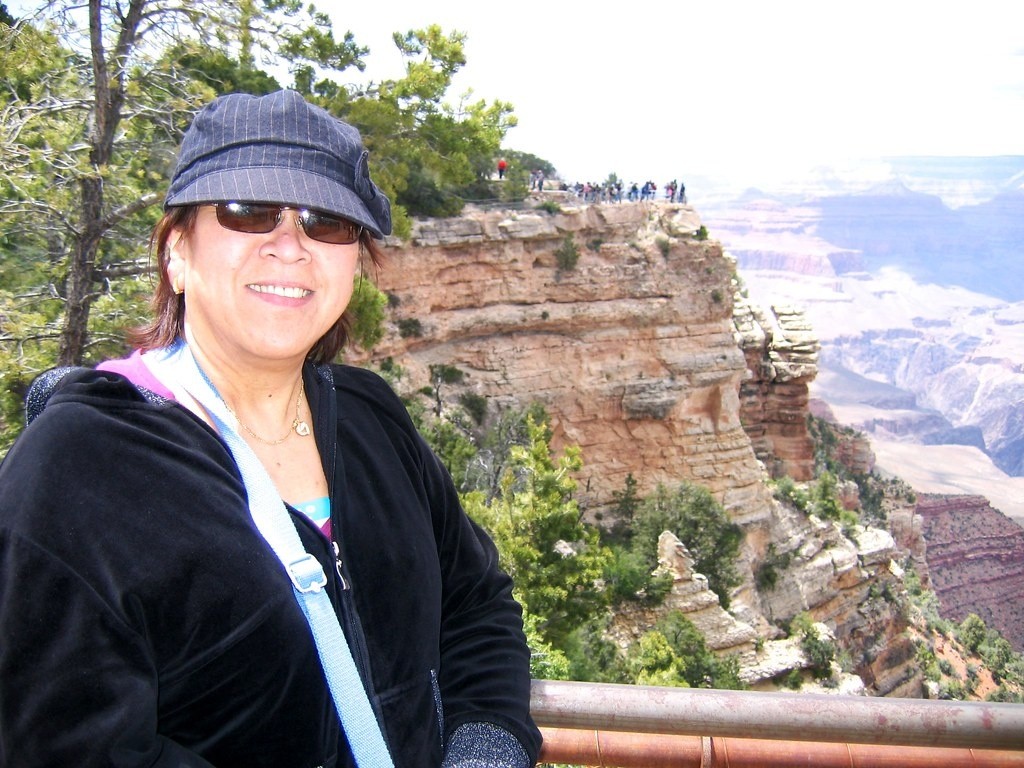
[231,379,310,446]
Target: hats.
[164,88,392,237]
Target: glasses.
[200,202,363,244]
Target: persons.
[0,90,545,767]
[498,157,506,179]
[530,170,543,191]
[564,179,686,205]
